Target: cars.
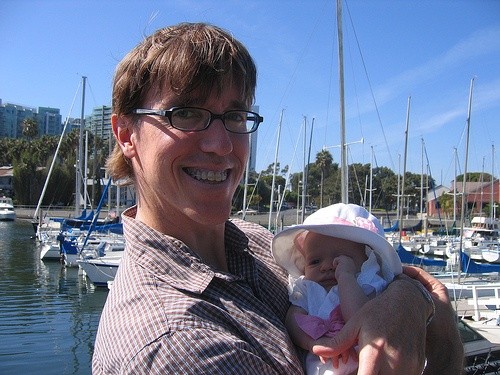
[280,202,294,211]
[399,207,419,216]
[237,208,258,216]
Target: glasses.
[132,105,264,134]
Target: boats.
[0,195,17,221]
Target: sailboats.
[32,0,499,374]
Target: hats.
[270,203,404,276]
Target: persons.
[272,202,403,375]
[92,22,465,375]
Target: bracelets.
[390,277,435,327]
